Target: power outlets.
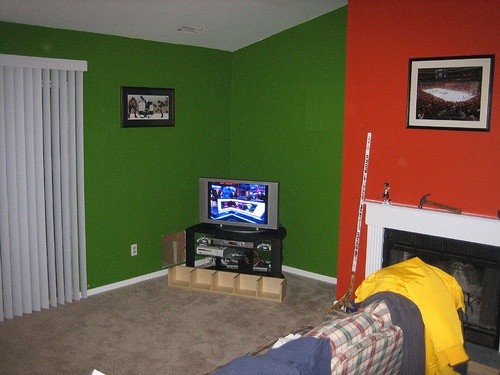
[131,244,137,256]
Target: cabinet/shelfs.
[168,266,287,303]
[186,223,287,279]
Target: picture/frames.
[120,85,175,128]
[405,54,494,131]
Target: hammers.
[417,193,461,214]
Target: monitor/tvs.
[199,178,280,234]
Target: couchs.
[205,301,403,375]
